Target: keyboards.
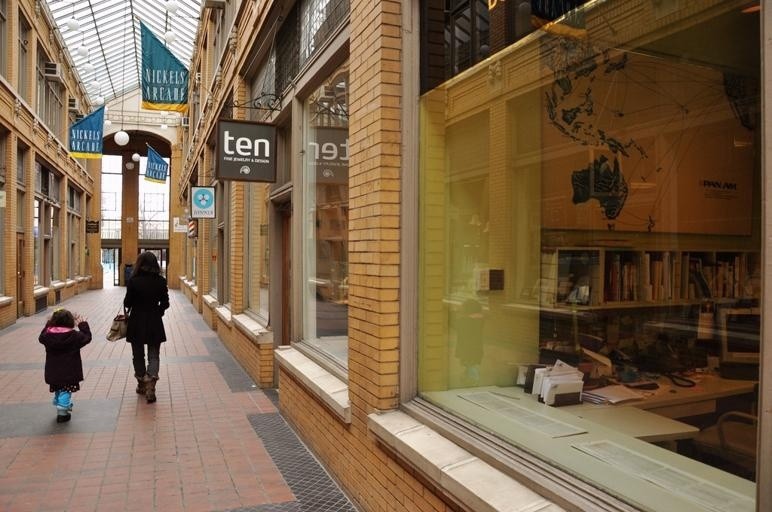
[720,361,759,381]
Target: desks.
[499,370,759,452]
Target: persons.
[38,308,93,423]
[123,252,170,403]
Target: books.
[557,250,760,303]
[515,306,715,407]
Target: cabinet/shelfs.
[541,226,760,314]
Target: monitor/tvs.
[719,305,761,364]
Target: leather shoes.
[57,413,71,423]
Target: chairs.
[691,398,757,473]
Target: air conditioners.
[45,61,65,84]
[195,73,201,87]
[182,117,189,128]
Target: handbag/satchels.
[106,301,133,341]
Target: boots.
[143,374,159,402]
[134,372,146,394]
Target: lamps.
[65,0,180,170]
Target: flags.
[66,107,106,159]
[139,20,190,113]
[144,146,170,184]
